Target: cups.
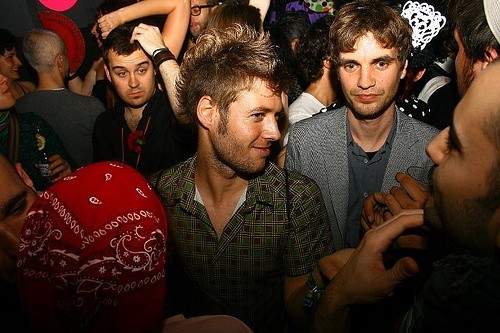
[407,165,434,192]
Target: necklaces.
[121,116,150,169]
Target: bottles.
[27,118,58,181]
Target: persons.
[1,0,500,333]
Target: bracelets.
[151,47,175,68]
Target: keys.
[303,272,323,310]
